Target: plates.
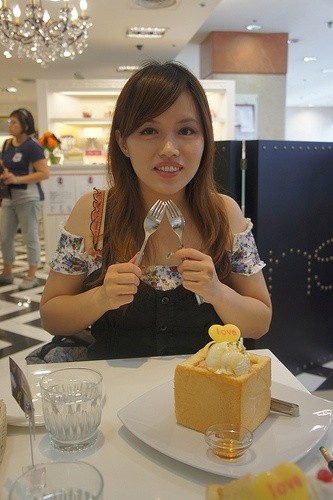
[117,377,333,478]
[0,364,106,426]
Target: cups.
[41,367,102,453]
[10,460,104,500]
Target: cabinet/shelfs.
[37,77,236,275]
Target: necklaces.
[163,249,176,261]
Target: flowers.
[37,131,61,157]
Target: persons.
[38,60,272,362]
[0,108,50,290]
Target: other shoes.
[18,275,39,290]
[0,272,15,285]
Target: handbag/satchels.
[0,138,13,199]
[24,326,95,365]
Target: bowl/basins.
[205,424,253,459]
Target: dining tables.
[0,350,333,500]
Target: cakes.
[174,324,272,435]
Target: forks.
[134,199,167,266]
[163,199,205,305]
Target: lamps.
[0,0,92,67]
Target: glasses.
[7,119,19,124]
